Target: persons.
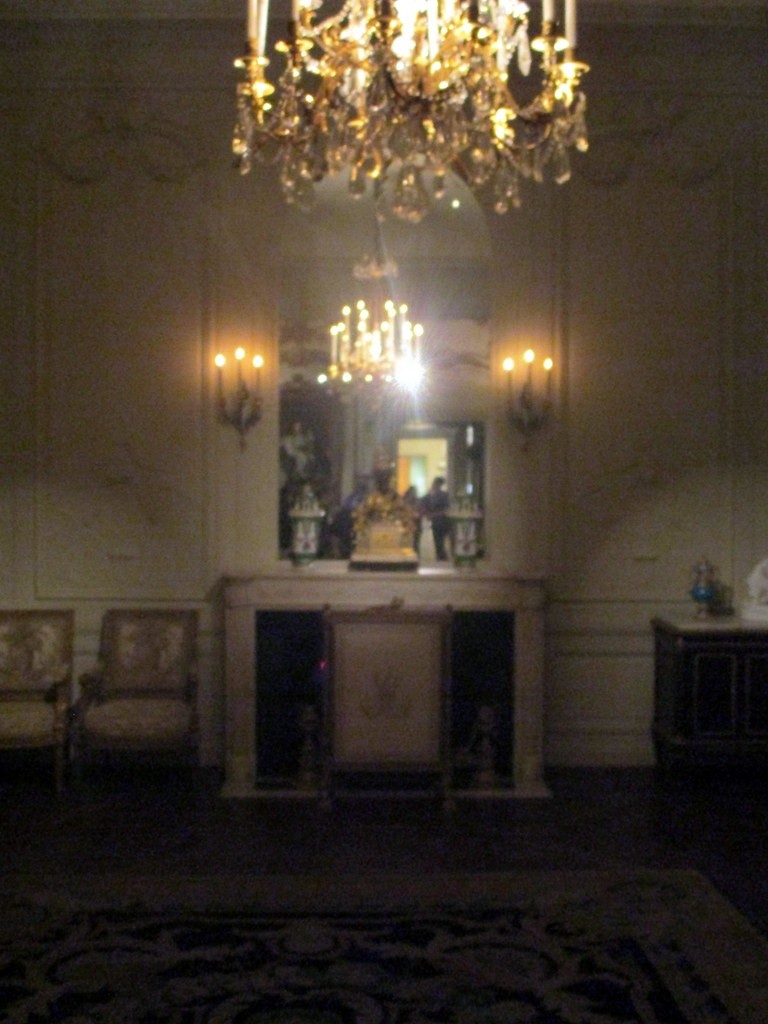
[278,453,456,561]
[688,560,714,622]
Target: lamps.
[315,227,426,387]
[232,0,594,224]
[213,347,267,453]
[501,347,557,453]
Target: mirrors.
[280,148,488,566]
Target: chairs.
[0,610,75,799]
[71,609,202,798]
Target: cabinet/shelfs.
[648,616,768,795]
